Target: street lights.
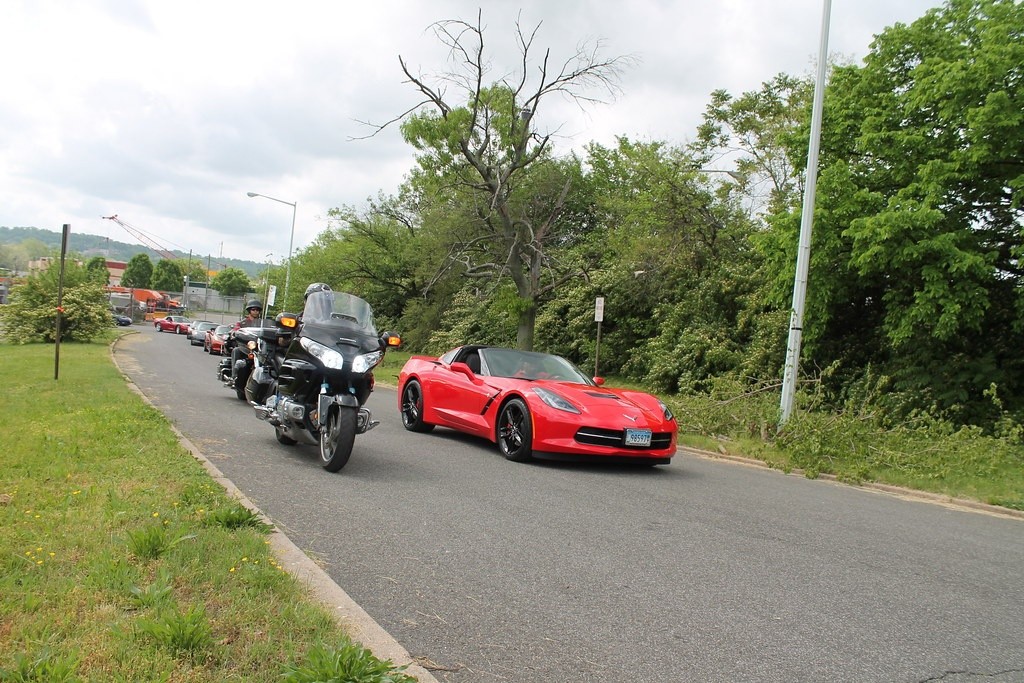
[247,192,297,311]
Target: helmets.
[245,300,263,311]
[303,283,334,301]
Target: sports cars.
[397,346,676,465]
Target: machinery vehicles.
[101,213,228,280]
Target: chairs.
[466,354,480,373]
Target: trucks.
[104,285,183,311]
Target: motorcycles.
[216,291,400,471]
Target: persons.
[511,357,559,380]
[224,299,266,387]
[278,283,374,414]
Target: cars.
[153,315,191,334]
[186,318,234,354]
[112,313,132,326]
